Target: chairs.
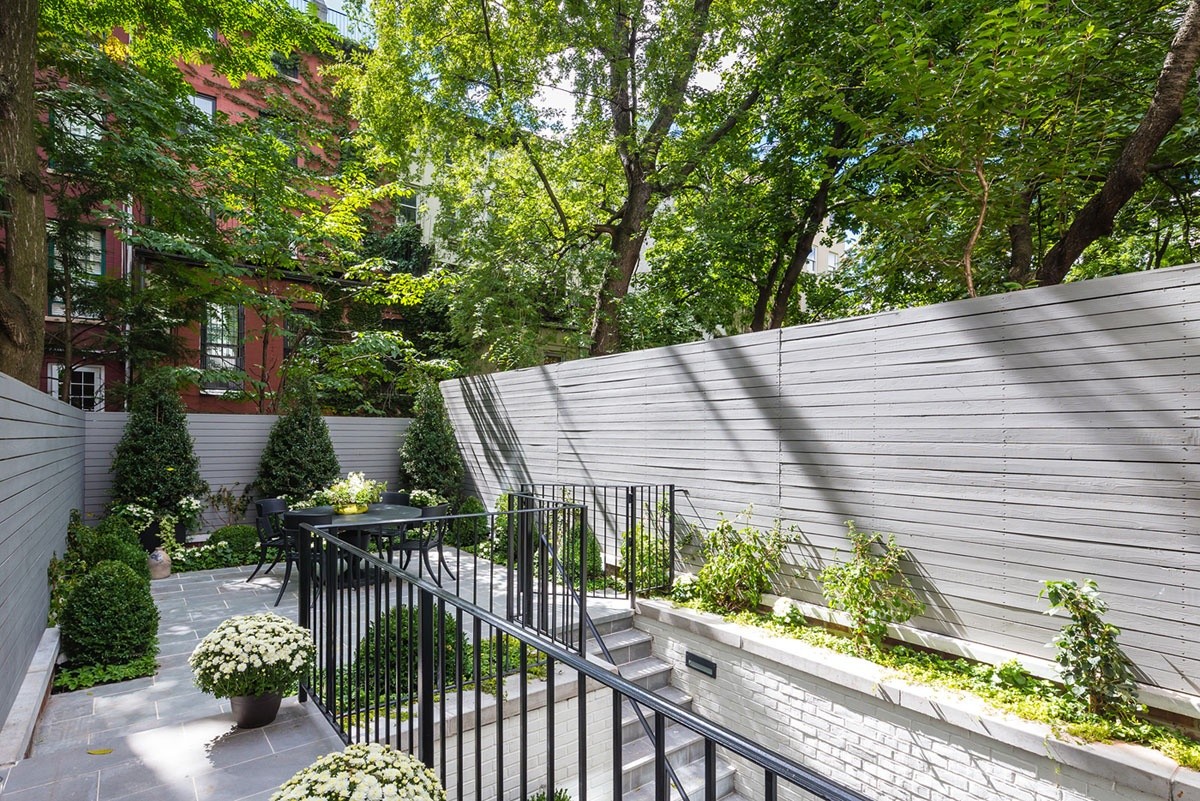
[368,491,456,588]
[246,497,357,609]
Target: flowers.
[186,611,316,699]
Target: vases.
[149,546,172,579]
[227,691,282,729]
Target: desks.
[294,504,423,589]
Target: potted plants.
[397,377,466,530]
[102,368,211,552]
